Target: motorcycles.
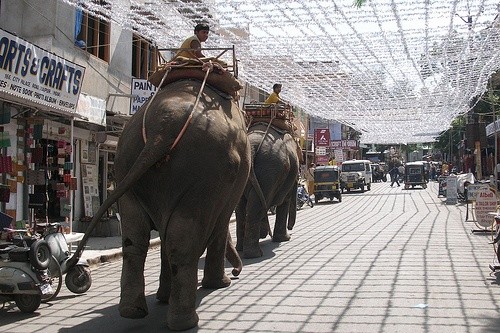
[296,179,313,210]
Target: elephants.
[235,120,304,261]
[389,158,401,182]
[66,75,252,330]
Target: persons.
[306,162,320,198]
[176,24,209,59]
[263,83,291,106]
[388,161,453,187]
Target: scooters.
[0,222,93,314]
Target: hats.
[273,84,282,88]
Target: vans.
[314,166,342,203]
[341,158,371,192]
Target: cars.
[371,161,429,190]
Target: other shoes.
[397,184,400,187]
[390,184,394,187]
